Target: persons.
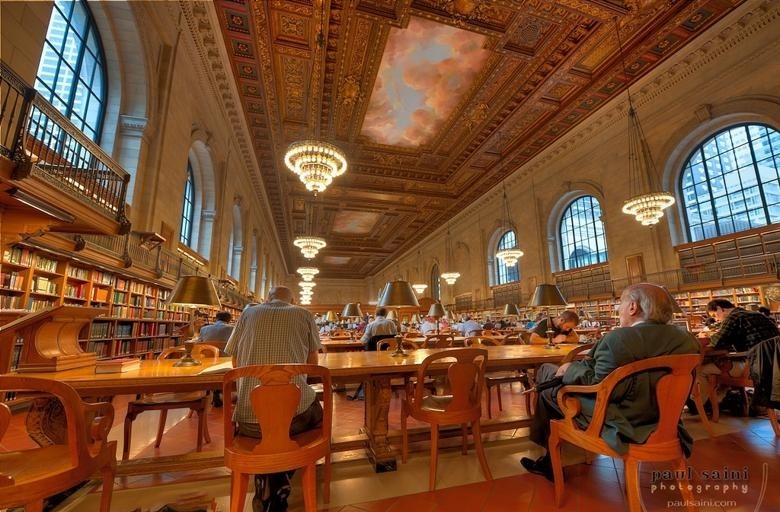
[319,308,546,350]
[527,310,579,343]
[195,313,236,410]
[519,283,701,484]
[701,299,779,418]
[223,285,323,438]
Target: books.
[0,243,241,401]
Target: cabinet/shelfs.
[0,230,243,369]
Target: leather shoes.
[520,456,565,481]
[267,472,292,512]
[252,474,270,512]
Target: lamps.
[411,208,428,293]
[284,0,348,197]
[318,282,587,356]
[613,17,675,228]
[495,135,525,267]
[439,188,460,285]
[294,198,327,305]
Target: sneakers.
[214,400,222,406]
